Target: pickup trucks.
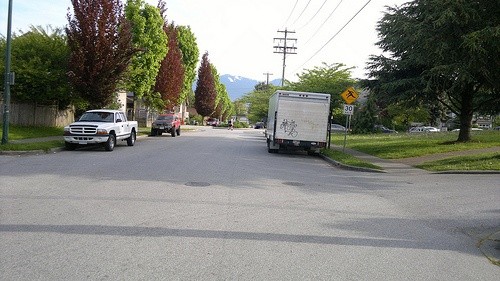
[63,110,139,151]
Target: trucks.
[264,89,331,156]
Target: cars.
[254,122,264,129]
[375,125,500,134]
[206,118,217,126]
[332,124,353,131]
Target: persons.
[228,119,233,130]
[259,120,265,130]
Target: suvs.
[151,114,181,137]
